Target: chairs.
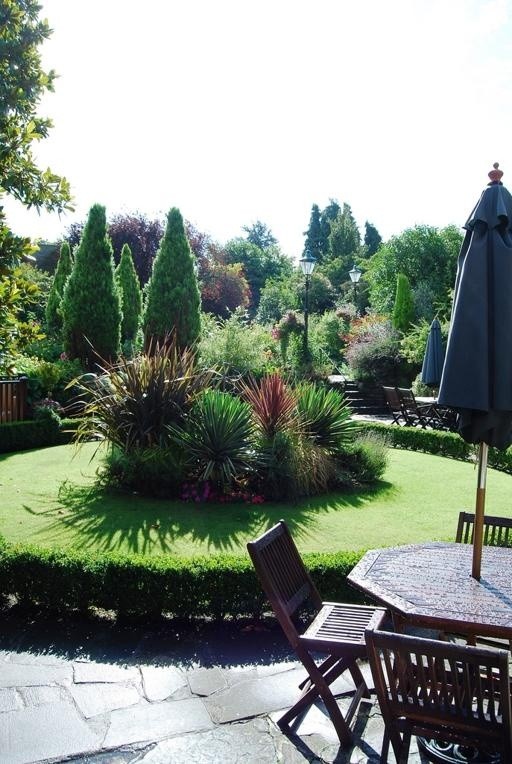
[363,628,511,764]
[456,512,512,546]
[249,518,391,747]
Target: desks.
[345,541,512,647]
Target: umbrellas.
[421,316,445,400]
[437,162,512,708]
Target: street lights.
[299,250,317,347]
[348,265,362,303]
